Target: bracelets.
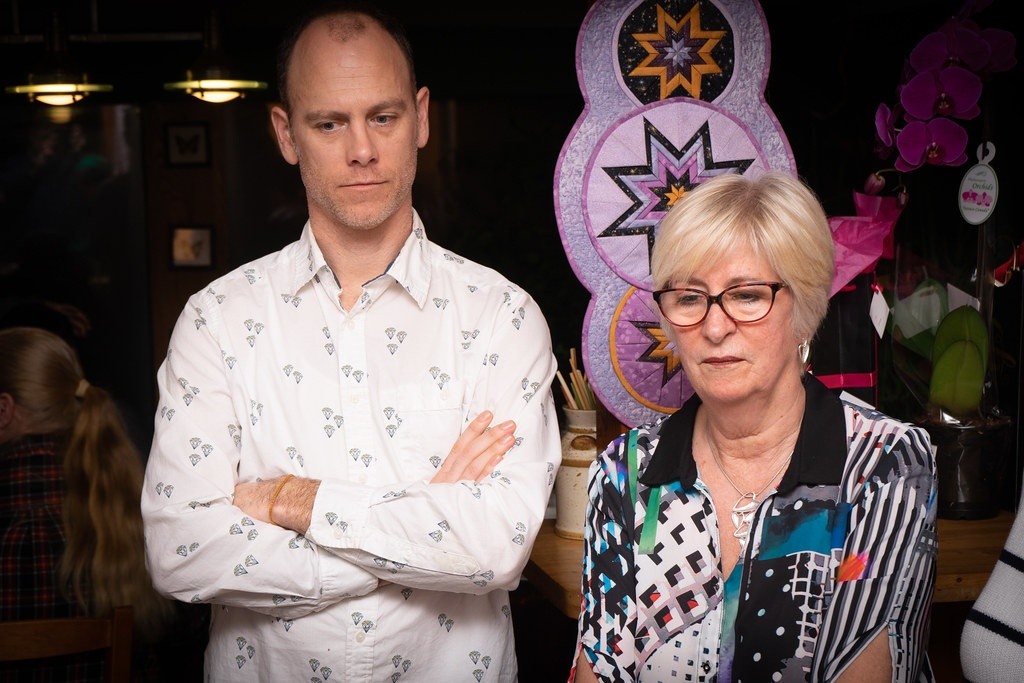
[267,473,297,525]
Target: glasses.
[653,283,790,326]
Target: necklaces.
[704,409,796,561]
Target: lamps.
[163,0,271,106]
[2,1,114,107]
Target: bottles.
[554,403,596,541]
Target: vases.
[882,163,1022,523]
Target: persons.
[959,469,1024,683]
[0,324,146,683]
[139,11,563,683]
[567,163,942,681]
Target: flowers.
[871,11,1023,418]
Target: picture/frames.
[166,221,217,273]
[162,119,214,169]
